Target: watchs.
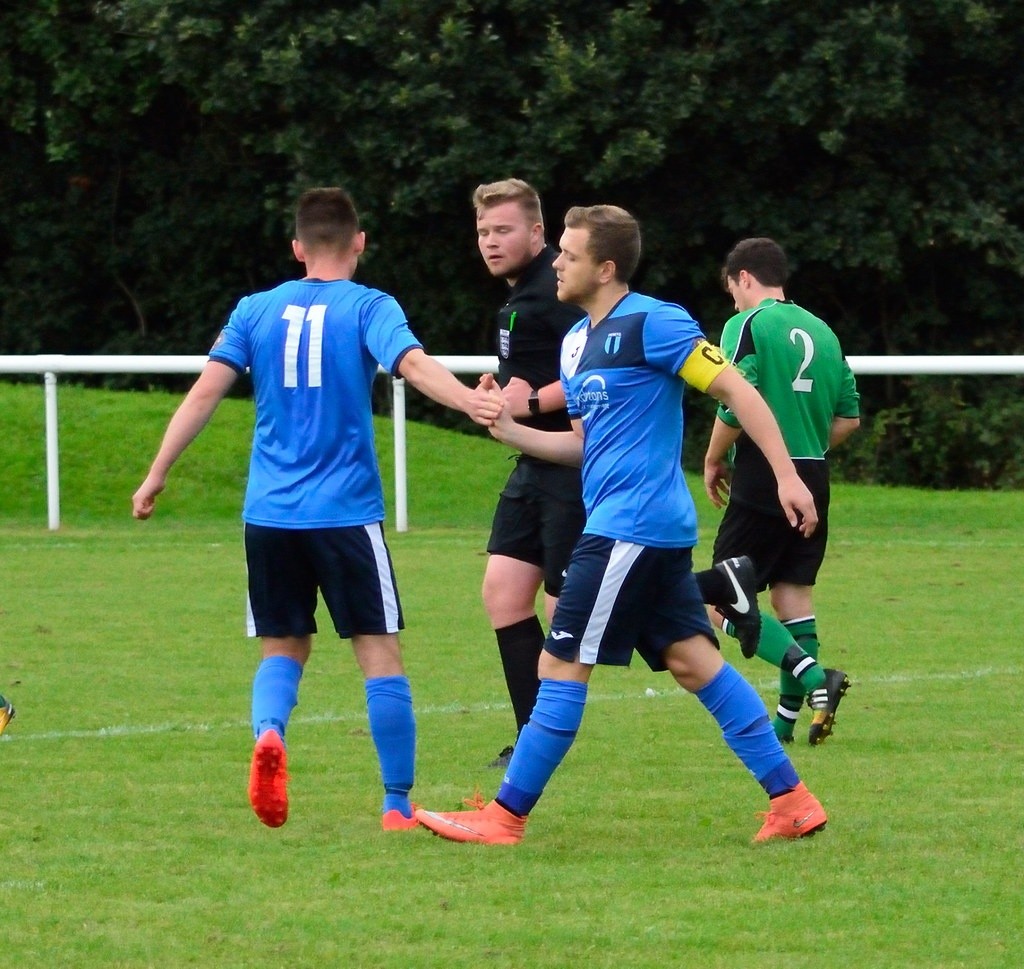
[527,389,541,416]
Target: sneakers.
[417,798,528,847]
[778,735,794,744]
[0,702,15,736]
[249,730,288,828]
[753,780,827,842]
[382,802,420,832]
[487,744,515,767]
[808,669,852,746]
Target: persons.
[470,178,764,771]
[702,237,862,748]
[128,187,503,831]
[415,205,831,847]
